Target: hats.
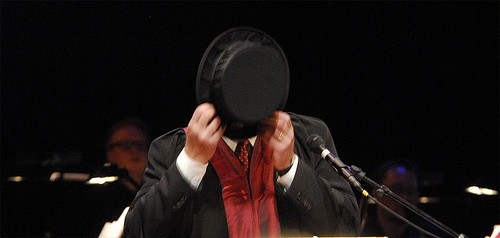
[192,24,291,139]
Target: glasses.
[108,139,146,152]
[386,182,420,196]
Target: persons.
[62,117,151,238]
[123,103,363,238]
[98,206,130,238]
[361,160,500,238]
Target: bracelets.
[277,166,292,177]
[126,175,142,189]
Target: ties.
[236,139,250,175]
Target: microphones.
[307,134,369,198]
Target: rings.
[278,131,285,142]
